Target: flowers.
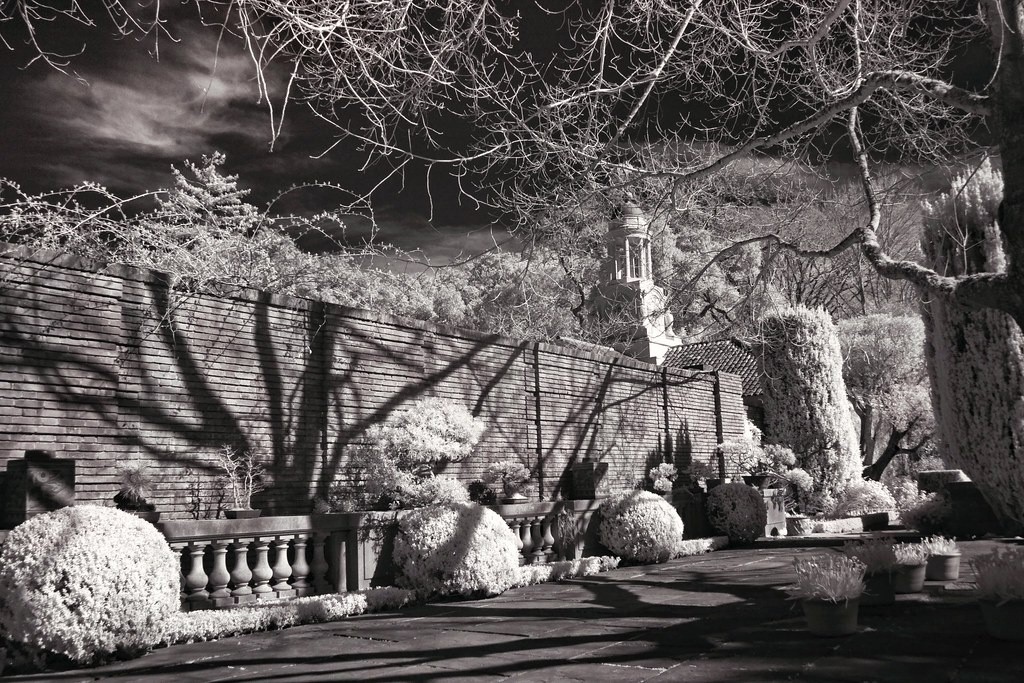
[888,536,930,567]
[484,460,533,500]
[921,534,962,557]
[113,461,165,510]
[785,553,870,609]
[844,530,904,579]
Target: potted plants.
[212,443,263,519]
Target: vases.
[927,554,962,579]
[860,575,896,624]
[801,596,862,636]
[893,562,927,593]
[501,496,531,504]
[124,510,160,524]
